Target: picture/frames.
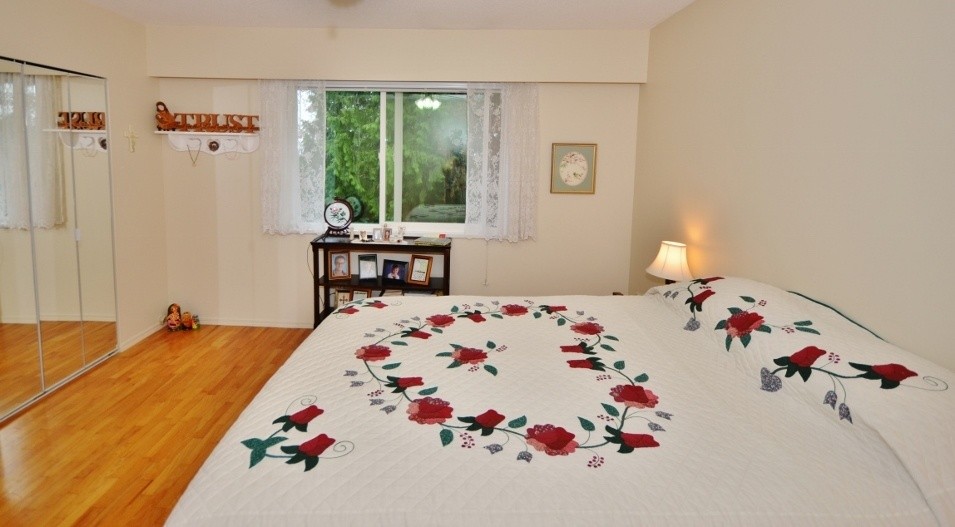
[382,228,393,241]
[381,258,409,284]
[334,287,352,311]
[350,285,372,302]
[407,255,433,285]
[328,250,351,280]
[358,253,377,280]
[373,228,382,241]
[550,143,598,195]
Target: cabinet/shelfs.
[311,233,452,330]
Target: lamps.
[646,240,695,286]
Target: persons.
[388,265,399,279]
[332,256,346,277]
[166,304,181,331]
[156,102,173,123]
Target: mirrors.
[0,55,121,424]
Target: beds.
[160,272,954,527]
